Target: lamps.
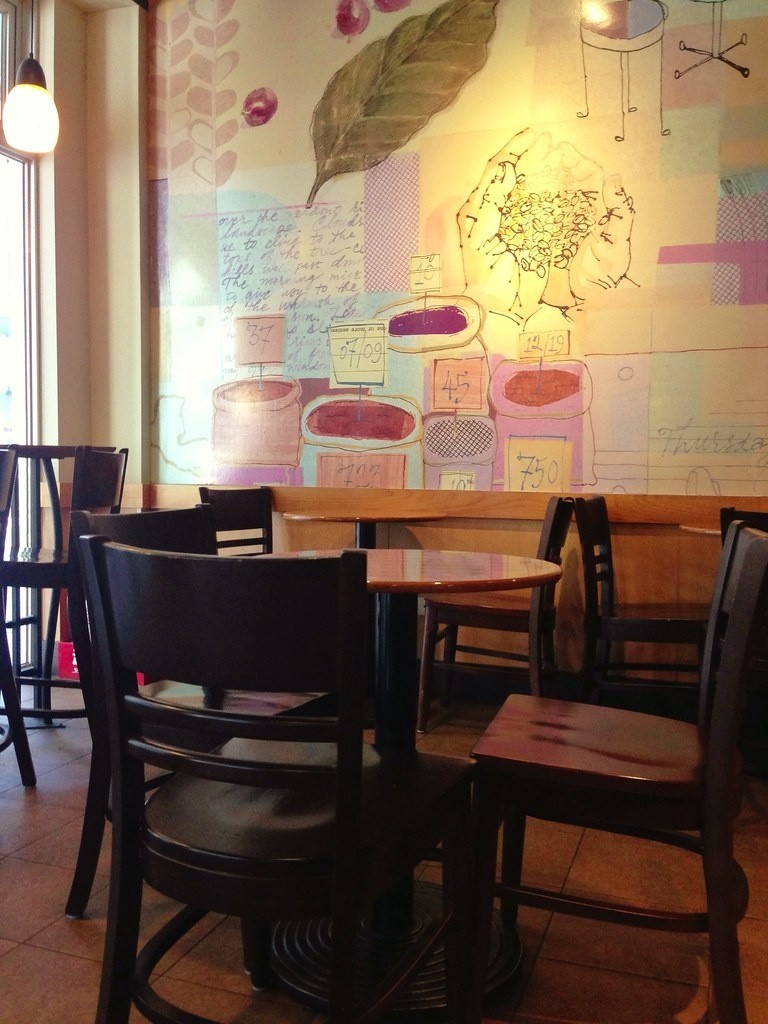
[2,0,60,154]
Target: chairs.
[199,486,272,557]
[417,496,577,733]
[65,502,333,923]
[721,506,768,779]
[468,516,768,1024]
[0,449,37,788]
[77,532,481,1024]
[0,445,130,719]
[573,496,713,705]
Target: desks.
[254,549,562,1024]
[285,508,450,728]
[678,519,722,537]
